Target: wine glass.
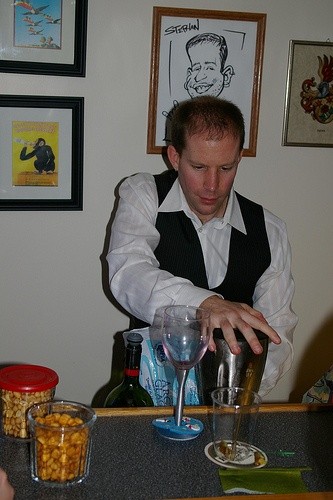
[156,304,211,441]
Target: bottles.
[102,332,154,408]
[200,328,268,408]
[0,364,59,444]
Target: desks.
[0,405,333,500]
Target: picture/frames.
[282,39,333,149]
[0,0,88,78]
[0,94,86,211]
[146,6,267,158]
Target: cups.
[27,400,98,490]
[212,386,261,460]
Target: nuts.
[0,387,53,439]
[32,411,88,482]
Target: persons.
[106,95,296,403]
